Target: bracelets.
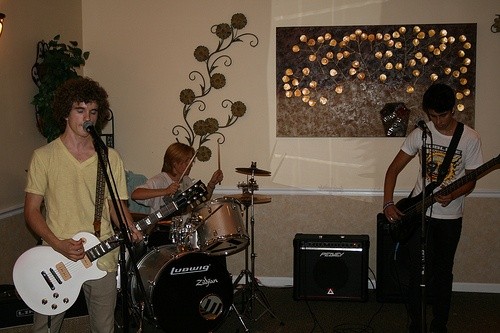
[208,183,215,189]
[383,201,394,212]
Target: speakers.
[292,232,369,302]
[376,212,409,306]
[0,284,89,333]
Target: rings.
[390,218,393,220]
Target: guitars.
[389,153,500,242]
[12,180,209,316]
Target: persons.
[131,143,223,214]
[24,75,145,333]
[382,84,483,333]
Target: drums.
[129,243,234,333]
[170,216,191,244]
[191,198,250,255]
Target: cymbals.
[236,168,272,174]
[224,195,271,204]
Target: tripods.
[231,171,287,326]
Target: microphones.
[414,117,432,135]
[83,121,108,151]
